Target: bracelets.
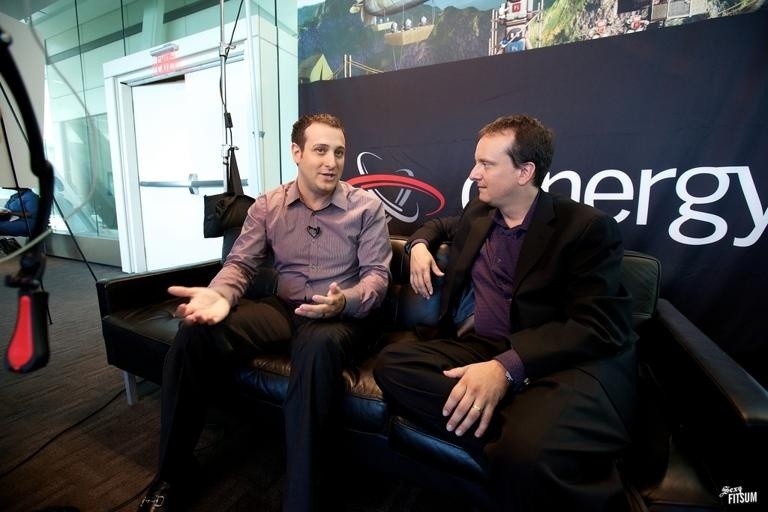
[340,295,346,313]
[504,370,513,382]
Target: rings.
[320,313,325,317]
[472,405,482,413]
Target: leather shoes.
[136,476,194,512]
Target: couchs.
[96,235,768,512]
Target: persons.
[372,110,635,512]
[134,110,395,511]
[0,188,40,235]
